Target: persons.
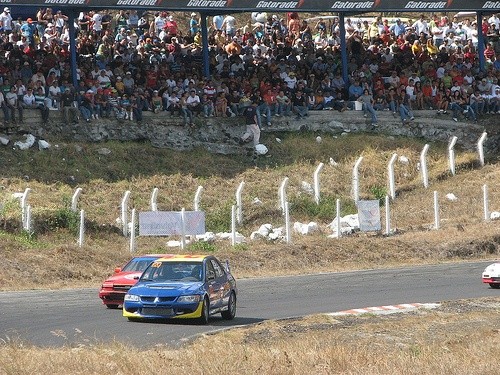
[0,7,74,123]
[240,99,260,148]
[469,12,500,116]
[396,90,414,123]
[191,265,202,279]
[451,90,468,121]
[357,89,378,127]
[74,10,208,127]
[204,12,351,117]
[61,88,80,123]
[460,90,478,123]
[347,12,475,115]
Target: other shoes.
[363,114,368,117]
[96,114,98,119]
[437,111,440,113]
[372,123,378,126]
[410,117,414,121]
[262,113,267,117]
[403,119,406,122]
[275,113,279,117]
[453,118,457,121]
[92,114,95,119]
[444,111,446,113]
[125,112,134,121]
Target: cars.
[99,253,174,309]
[481,263,500,289]
[123,254,238,326]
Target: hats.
[4,7,11,13]
[445,89,450,93]
[117,76,122,80]
[169,15,174,21]
[126,72,131,75]
